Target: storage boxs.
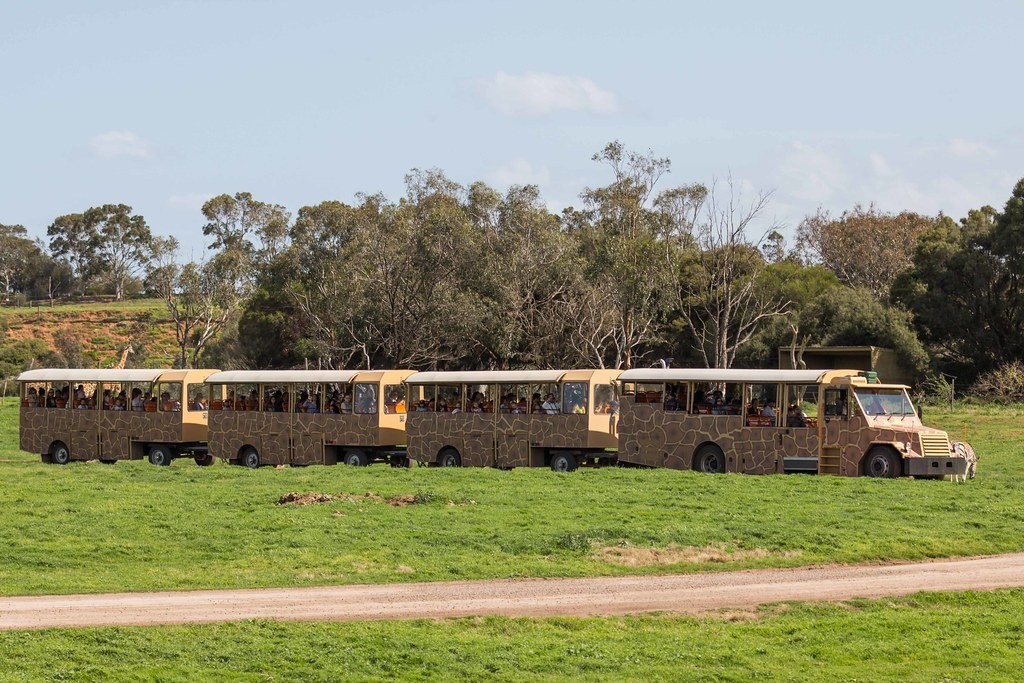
[858,371,878,384]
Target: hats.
[119,390,126,397]
[196,393,203,397]
[507,393,516,399]
[519,397,527,403]
[455,403,461,407]
[39,387,45,394]
[420,400,425,404]
[532,393,541,399]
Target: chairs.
[636,387,832,431]
[21,396,207,412]
[409,397,569,416]
[210,398,400,414]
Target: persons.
[223,383,406,414]
[27,384,208,412]
[571,397,587,414]
[871,396,885,413]
[660,384,806,427]
[416,390,611,414]
[748,398,758,415]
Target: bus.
[17,366,967,480]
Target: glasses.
[795,408,800,410]
[140,394,143,395]
[49,392,54,393]
[391,394,398,396]
[538,398,541,399]
[200,397,203,398]
[163,395,168,397]
[32,392,36,394]
